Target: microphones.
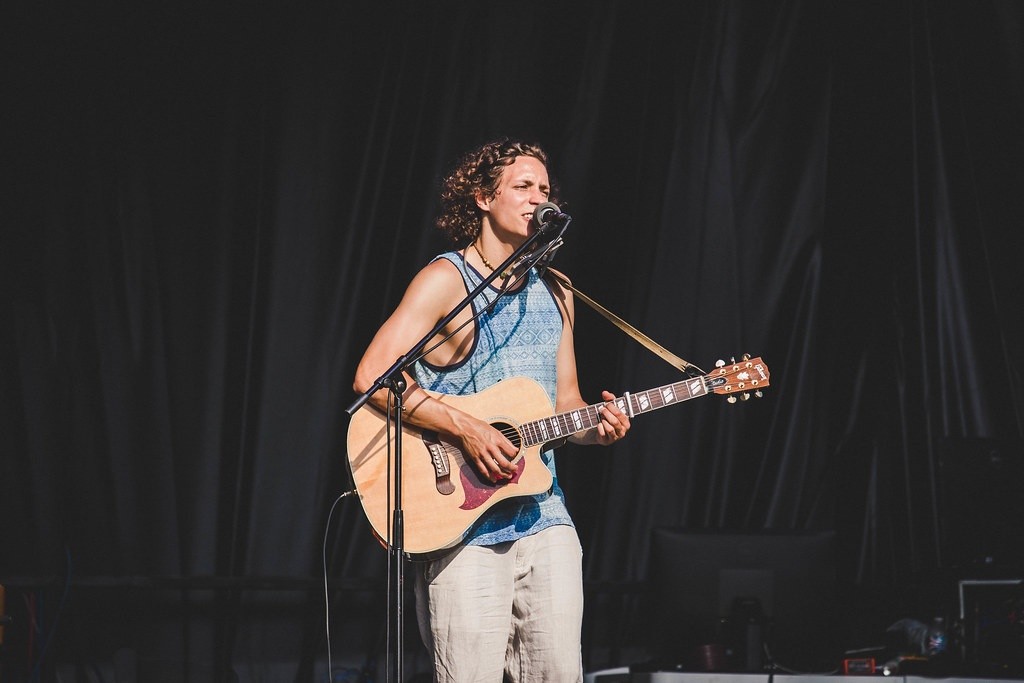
[533,202,572,232]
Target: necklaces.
[473,243,508,279]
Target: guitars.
[342,352,772,562]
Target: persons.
[353,137,631,683]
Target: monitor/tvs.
[641,526,839,662]
